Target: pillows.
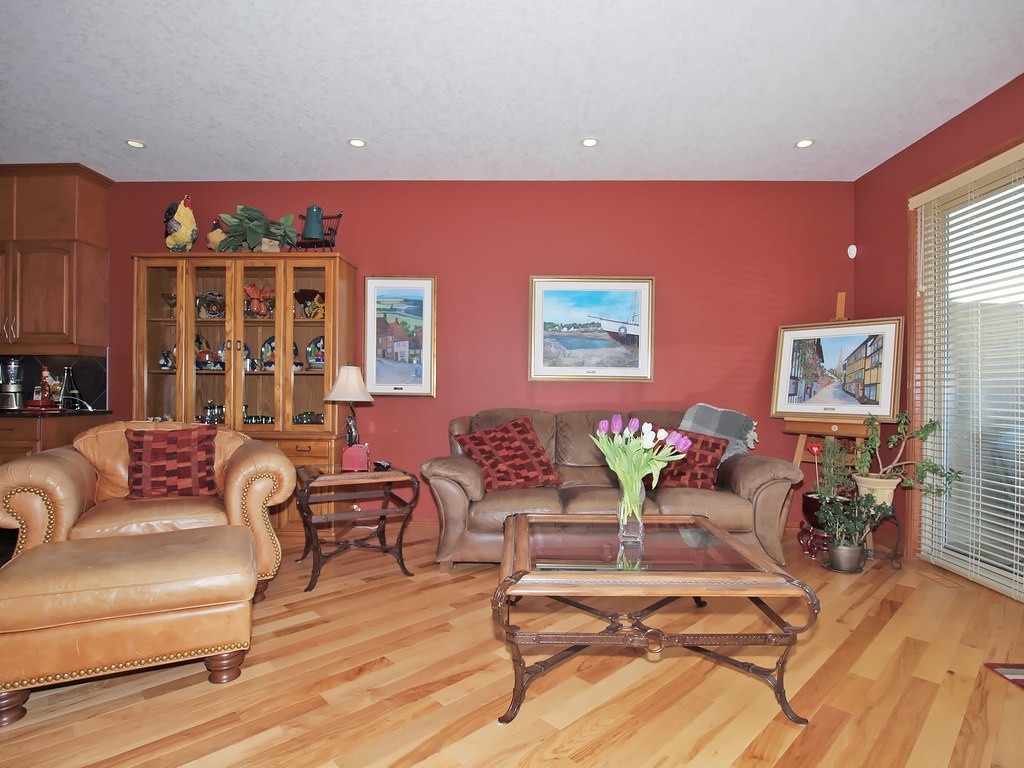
[679,403,759,469]
[641,422,729,491]
[453,415,562,493]
[124,424,223,501]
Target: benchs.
[0,525,258,727]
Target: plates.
[173,333,324,364]
[200,290,225,318]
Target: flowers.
[586,413,692,528]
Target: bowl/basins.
[196,359,324,371]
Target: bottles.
[59,366,81,410]
[243,296,274,319]
[159,350,172,370]
[148,399,324,424]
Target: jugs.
[304,203,324,240]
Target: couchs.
[420,407,804,574]
[0,419,296,604]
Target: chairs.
[289,212,342,252]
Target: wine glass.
[160,293,177,319]
[195,294,207,319]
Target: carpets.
[983,662,1024,690]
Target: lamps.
[322,362,375,470]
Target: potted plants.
[795,412,965,573]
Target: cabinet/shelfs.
[130,251,357,538]
[0,162,116,468]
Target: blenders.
[0,358,27,412]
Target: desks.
[294,459,420,592]
[490,512,821,723]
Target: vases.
[616,478,645,542]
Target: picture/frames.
[361,274,437,399]
[528,274,654,383]
[769,315,906,423]
